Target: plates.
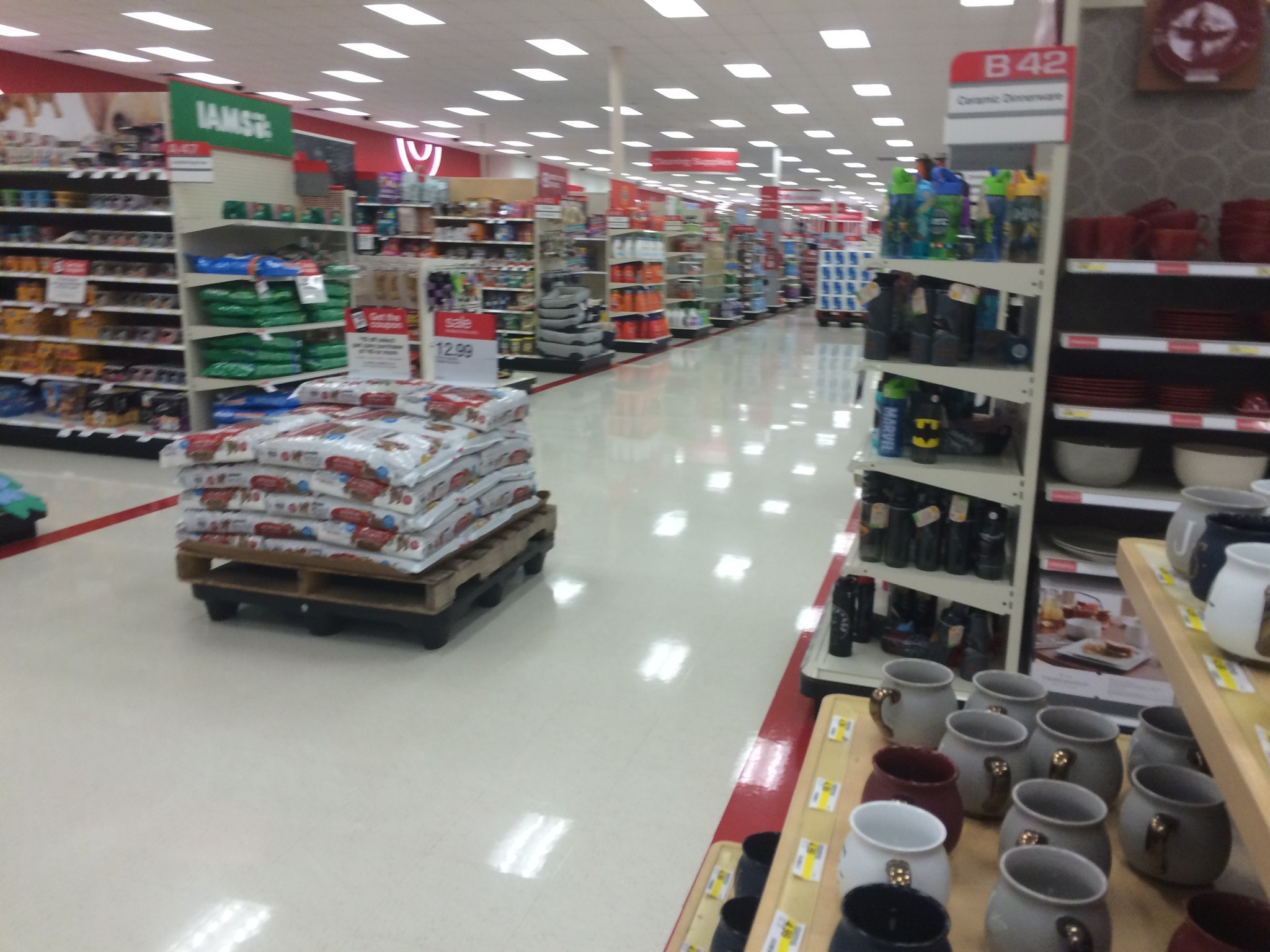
[1159,307,1237,340]
[1057,637,1152,671]
[1049,525,1134,562]
[1048,374,1217,414]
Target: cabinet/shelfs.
[1,83,819,459]
[802,0,1270,732]
[744,536,1270,951]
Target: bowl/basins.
[1055,439,1141,487]
[1066,618,1101,640]
[1123,617,1149,648]
[1216,198,1270,261]
[1173,443,1269,490]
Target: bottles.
[878,381,908,458]
[858,478,1007,581]
[829,572,992,681]
[879,165,1047,264]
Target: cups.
[1165,479,1270,667]
[712,658,1270,952]
[1065,197,1211,261]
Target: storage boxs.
[816,250,878,312]
